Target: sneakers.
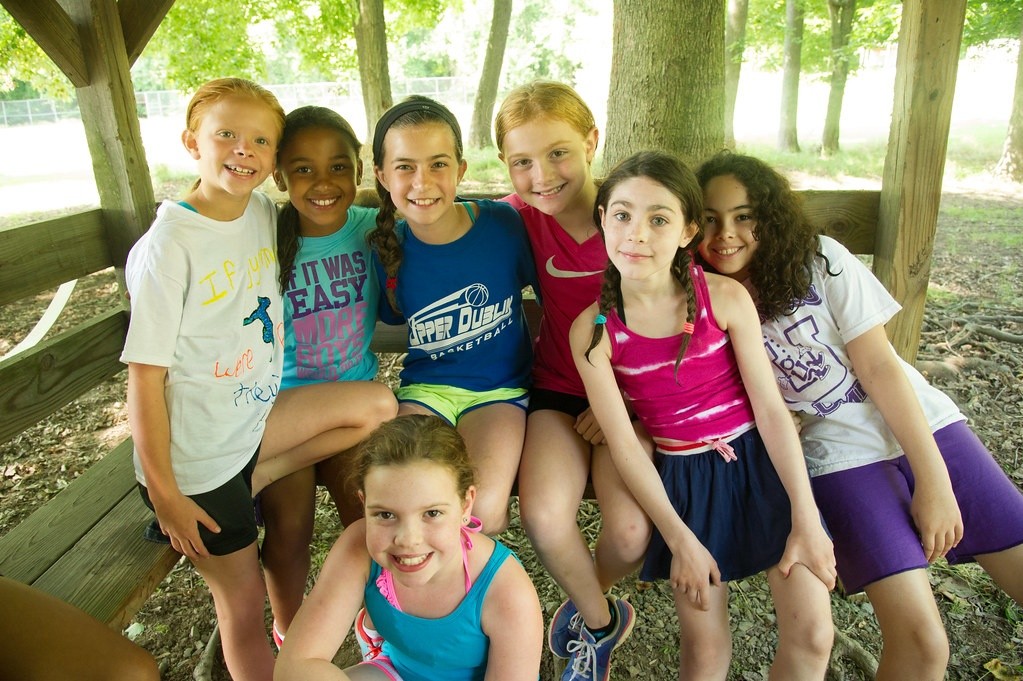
[355,606,385,661]
[559,594,635,681]
[548,585,612,660]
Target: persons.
[120,76,398,679]
[567,148,836,677]
[370,94,544,533]
[272,415,543,681]
[247,102,429,659]
[685,155,1023,680]
[494,81,653,679]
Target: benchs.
[0,189,879,631]
